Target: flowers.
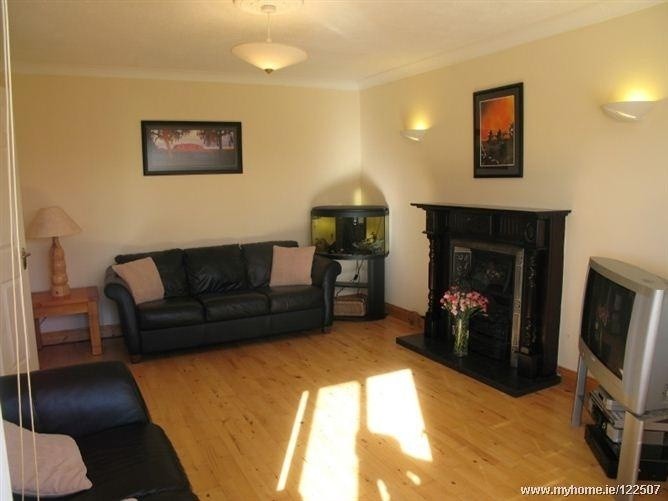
[439,283,489,348]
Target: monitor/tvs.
[578,255,667,417]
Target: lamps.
[399,129,429,142]
[230,0,308,74]
[599,101,658,120]
[26,204,84,298]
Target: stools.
[30,284,105,356]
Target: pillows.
[3,420,92,498]
[111,256,164,305]
[269,245,315,287]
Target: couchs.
[104,241,342,364]
[0,361,201,501]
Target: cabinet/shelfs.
[573,357,668,483]
[333,257,386,321]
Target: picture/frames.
[473,82,523,178]
[140,120,243,176]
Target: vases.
[449,318,472,357]
